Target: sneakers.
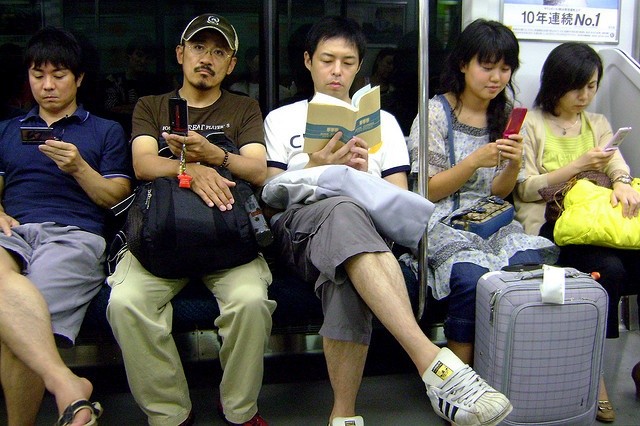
[235,413,269,426]
[596,399,614,422]
[421,346,513,426]
[328,415,364,426]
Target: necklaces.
[547,112,582,135]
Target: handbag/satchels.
[450,195,514,239]
[553,176,640,250]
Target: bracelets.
[217,146,229,169]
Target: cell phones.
[20,126,55,145]
[501,108,529,138]
[603,126,633,151]
[167,97,188,135]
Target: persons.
[263,15,513,426]
[514,41,640,424]
[408,17,564,426]
[102,18,279,425]
[1,26,134,426]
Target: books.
[301,83,386,158]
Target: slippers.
[58,398,103,425]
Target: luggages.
[473,262,609,426]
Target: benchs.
[54,258,419,368]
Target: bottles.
[247,193,273,247]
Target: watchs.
[609,174,633,187]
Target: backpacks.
[104,129,274,277]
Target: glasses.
[184,40,232,60]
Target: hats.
[537,170,612,223]
[180,13,239,56]
